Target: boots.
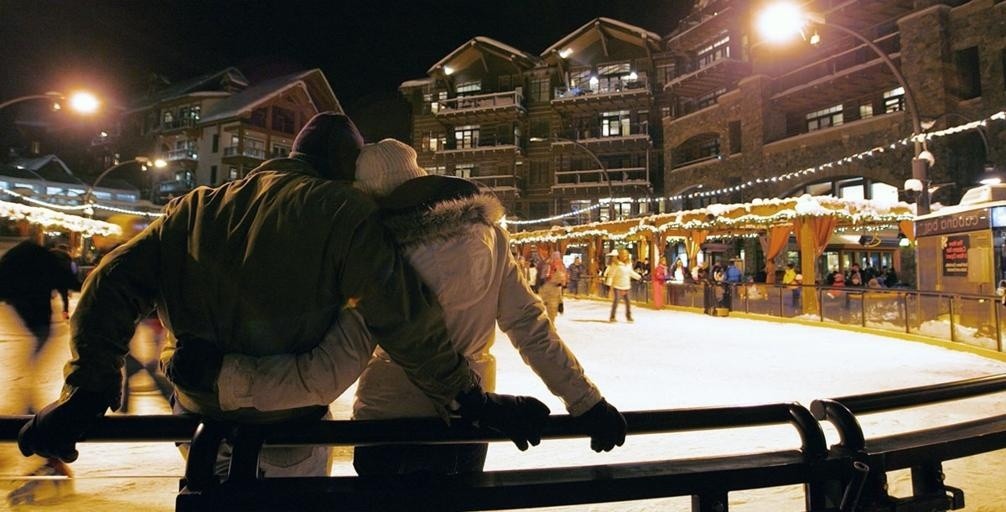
[609,311,635,322]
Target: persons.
[651,256,743,309]
[518,246,584,323]
[602,249,650,323]
[821,261,899,301]
[0,215,150,351]
[18,114,550,477]
[173,138,629,475]
[781,262,801,309]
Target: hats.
[287,111,429,197]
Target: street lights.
[752,1,931,216]
[0,89,102,115]
[16,165,48,194]
[920,109,994,187]
[84,157,168,200]
[530,136,615,253]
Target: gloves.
[458,385,551,451]
[17,392,98,463]
[167,332,223,392]
[573,398,628,452]
